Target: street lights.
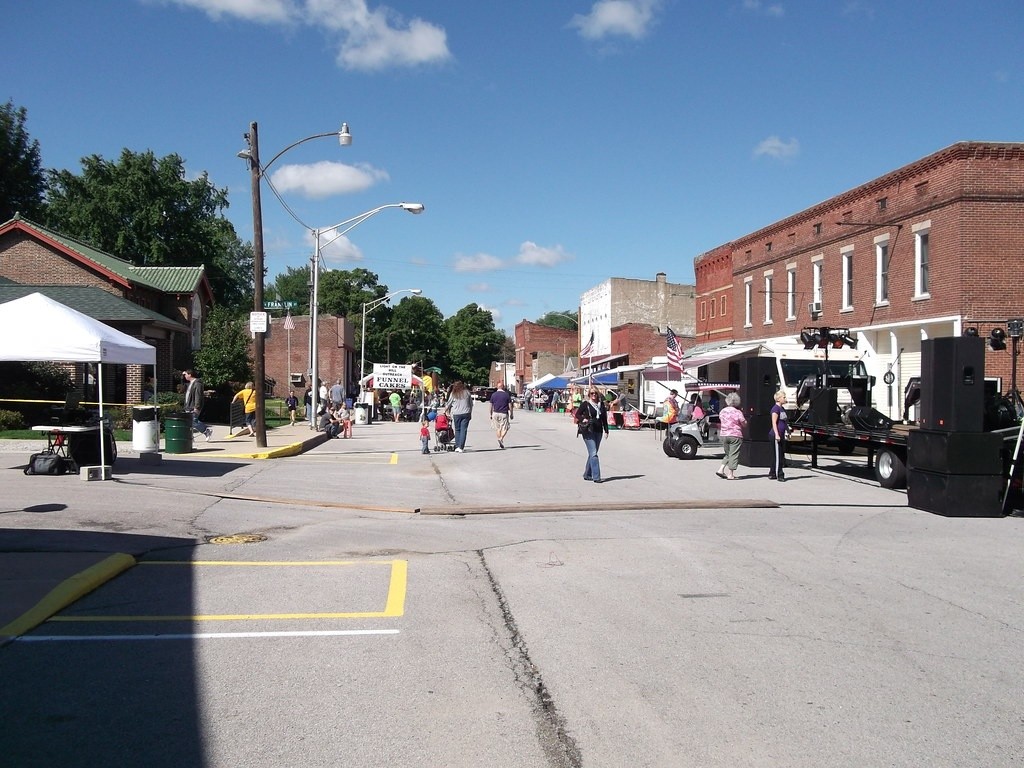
[386,329,415,364]
[412,349,430,364]
[310,201,425,429]
[237,122,353,449]
[360,289,422,388]
[486,342,507,390]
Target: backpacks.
[343,419,352,439]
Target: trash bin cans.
[355,403,372,425]
[164,413,193,453]
[132,406,159,453]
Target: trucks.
[691,345,876,455]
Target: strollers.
[434,413,457,451]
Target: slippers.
[716,471,727,479]
[727,476,739,480]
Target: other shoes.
[497,439,505,448]
[594,480,602,483]
[290,422,294,426]
[308,422,312,426]
[330,435,339,439]
[205,429,213,442]
[777,477,785,482]
[248,432,254,437]
[584,478,593,480]
[422,449,430,454]
[455,447,464,452]
[768,475,778,479]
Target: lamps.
[800,328,859,350]
[961,321,1007,350]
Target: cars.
[478,388,517,403]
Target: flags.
[667,325,684,373]
[579,332,595,359]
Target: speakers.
[738,355,777,468]
[848,406,894,431]
[905,336,1007,518]
[810,387,837,425]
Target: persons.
[231,381,256,437]
[434,380,473,452]
[419,420,431,454]
[716,392,748,480]
[362,385,442,423]
[303,380,351,439]
[662,390,719,437]
[285,391,298,426]
[575,386,609,483]
[521,388,587,417]
[183,368,213,442]
[609,390,627,411]
[489,383,513,449]
[144,377,157,405]
[768,391,791,481]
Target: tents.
[358,363,433,403]
[527,368,618,411]
[0,292,159,481]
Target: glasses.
[591,392,597,395]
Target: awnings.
[641,345,762,404]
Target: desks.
[32,424,113,469]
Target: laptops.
[53,392,82,410]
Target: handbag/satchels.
[577,402,594,437]
[24,450,67,476]
